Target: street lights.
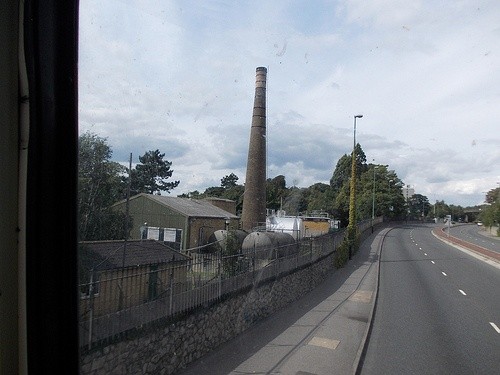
[387,179,394,229]
[346,114,363,260]
[371,164,380,234]
